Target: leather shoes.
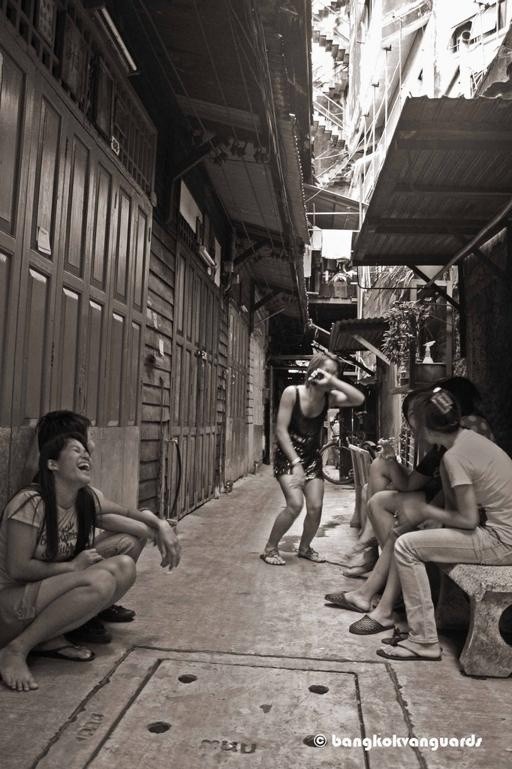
[100,604,135,620]
[65,618,111,644]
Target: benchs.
[431,561,512,678]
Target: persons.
[375,383,511,664]
[29,407,184,646]
[253,353,366,566]
[1,432,183,692]
[340,390,448,577]
[321,374,498,636]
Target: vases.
[413,362,446,389]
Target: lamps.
[87,4,143,77]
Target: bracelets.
[288,457,302,468]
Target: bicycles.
[318,410,378,485]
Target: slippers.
[376,638,442,660]
[381,628,408,644]
[297,547,326,563]
[349,613,395,635]
[325,590,367,612]
[260,547,286,565]
[33,644,97,661]
